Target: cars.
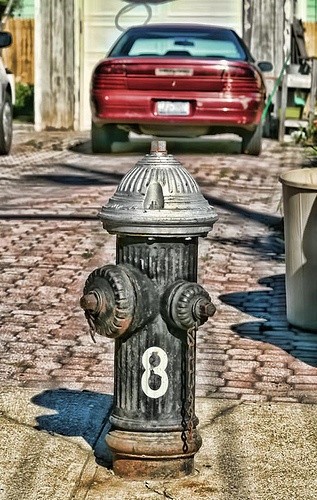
[89,23,266,156]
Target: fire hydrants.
[78,137,218,481]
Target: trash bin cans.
[278,168,317,331]
